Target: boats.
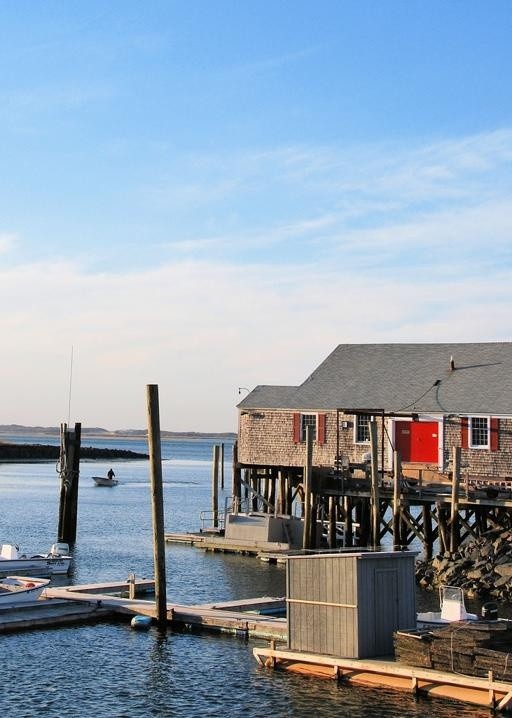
[0,575,50,603]
[416,584,512,637]
[92,476,117,485]
[0,541,72,578]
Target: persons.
[108,469,115,479]
